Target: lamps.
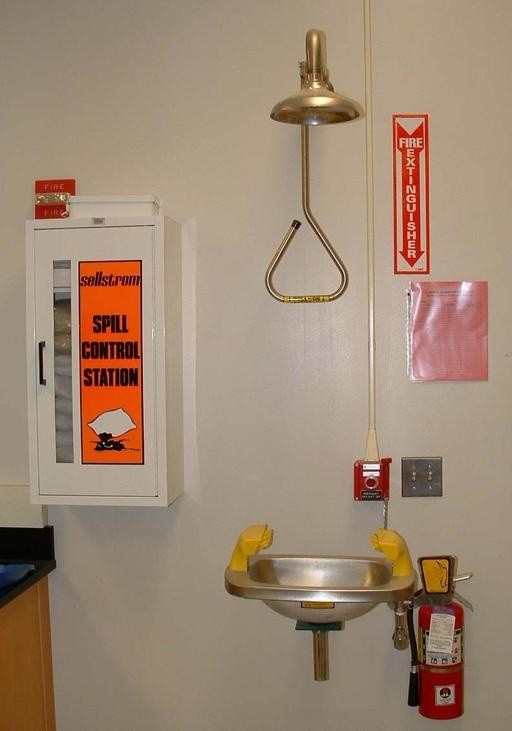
[264,28,363,305]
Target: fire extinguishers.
[408,573,475,720]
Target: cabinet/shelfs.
[23,192,183,508]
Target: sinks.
[225,554,417,623]
